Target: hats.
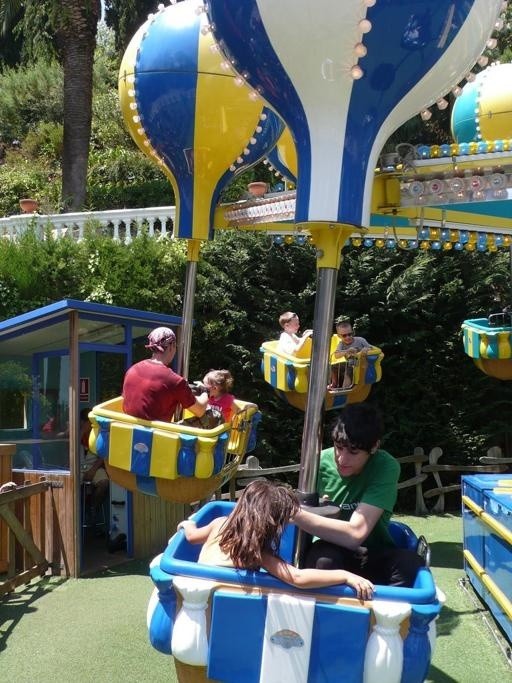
[144,327,176,352]
[332,403,399,449]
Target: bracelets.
[202,388,210,397]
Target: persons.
[43,410,69,438]
[289,403,418,588]
[80,408,110,525]
[487,294,511,323]
[203,369,259,432]
[177,480,377,600]
[277,311,313,355]
[122,327,210,423]
[327,321,372,388]
